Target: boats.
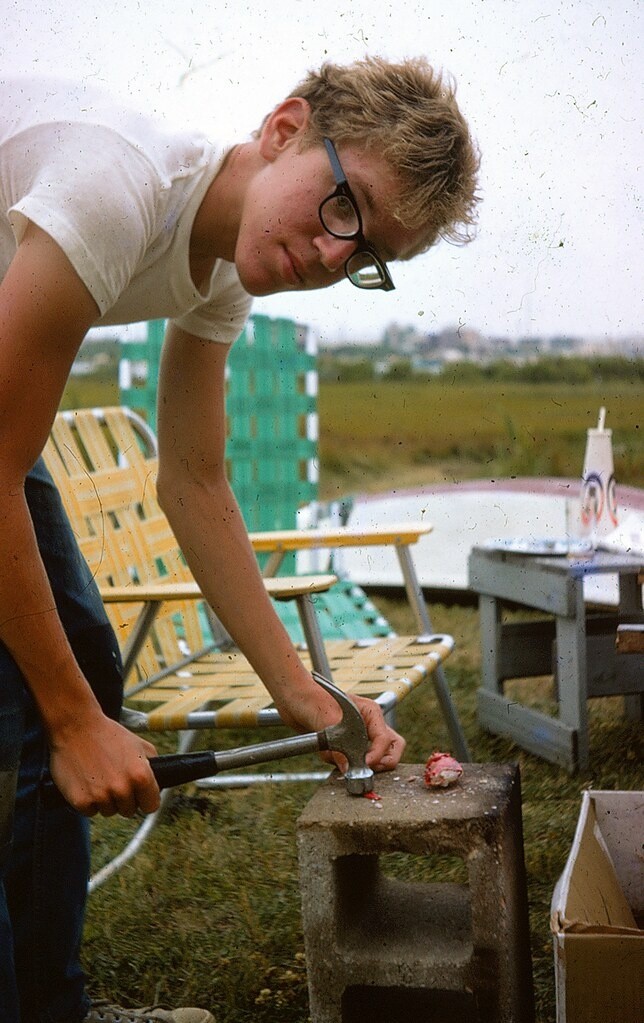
[288,472,644,619]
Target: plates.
[495,544,568,556]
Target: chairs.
[118,311,401,785]
[42,407,467,889]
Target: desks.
[468,548,644,780]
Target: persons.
[0,54,484,1023]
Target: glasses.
[318,135,396,292]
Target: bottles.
[584,429,617,544]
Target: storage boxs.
[549,788,644,1023]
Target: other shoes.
[82,998,216,1023]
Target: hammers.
[40,671,376,812]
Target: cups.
[565,496,596,565]
